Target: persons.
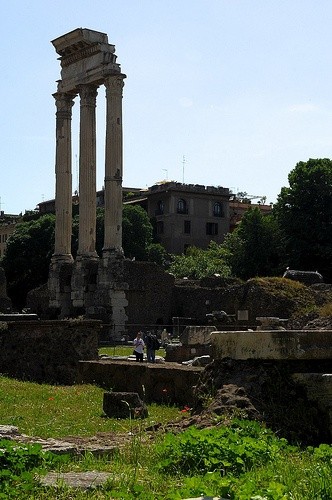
[145,331,158,363]
[133,331,146,362]
